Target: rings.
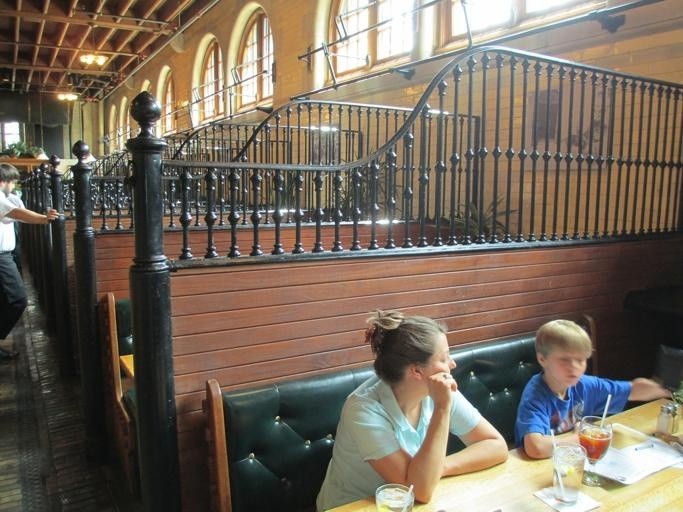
[443,375,448,380]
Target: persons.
[514,318,672,458]
[0,162,59,359]
[314,309,508,512]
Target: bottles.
[657,403,681,435]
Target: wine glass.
[578,415,613,487]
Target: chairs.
[93,290,139,503]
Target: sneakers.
[1,347,21,359]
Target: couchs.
[201,314,600,511]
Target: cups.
[375,483,415,512]
[551,440,587,506]
